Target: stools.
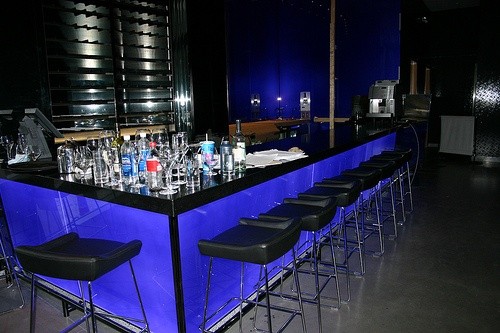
[198,149,414,333]
[14,232,151,333]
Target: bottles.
[136,132,150,183]
[147,142,164,191]
[120,134,139,190]
[233,119,246,173]
[220,137,233,175]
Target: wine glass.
[203,148,219,176]
[74,127,191,195]
[0,133,42,166]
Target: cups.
[222,147,234,174]
[90,149,110,184]
[185,152,201,187]
[199,141,215,175]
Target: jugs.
[56,139,76,174]
